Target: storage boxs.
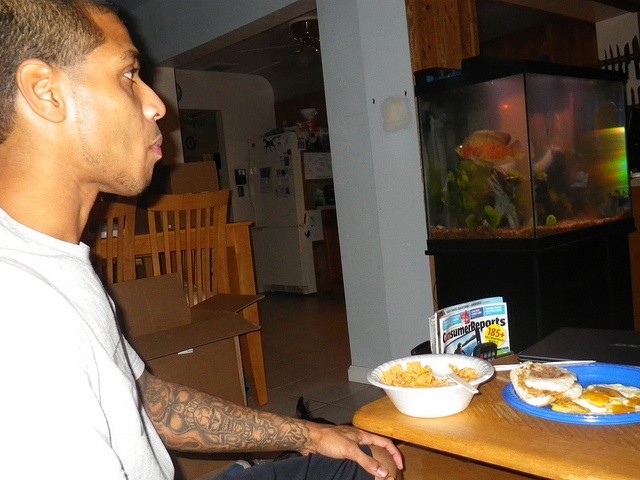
[101,273,266,475]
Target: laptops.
[517,326,640,367]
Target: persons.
[0,1,405,480]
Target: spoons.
[432,363,479,396]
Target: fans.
[240,15,321,79]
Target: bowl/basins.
[367,353,496,419]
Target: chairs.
[147,190,231,305]
[85,202,136,289]
[145,160,222,304]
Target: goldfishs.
[454,131,524,168]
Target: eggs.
[571,383,640,416]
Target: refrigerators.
[247,128,338,296]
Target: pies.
[526,363,577,394]
[508,364,562,408]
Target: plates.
[502,361,636,427]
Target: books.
[428,315,436,354]
[434,296,504,355]
[439,302,511,358]
